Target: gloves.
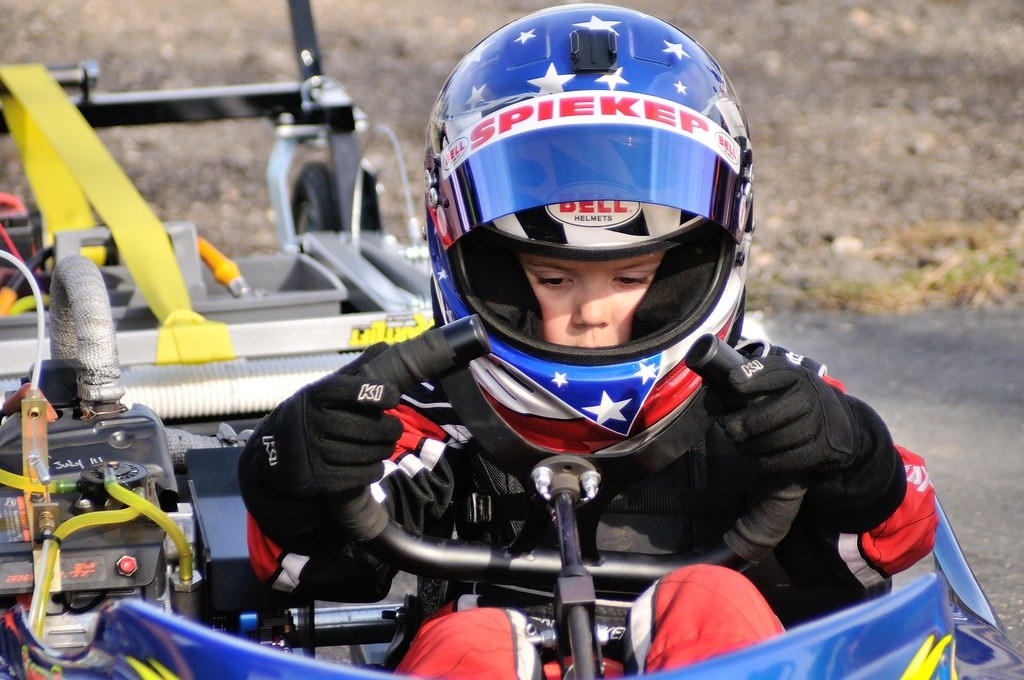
[705,355,859,488]
[238,341,406,556]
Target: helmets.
[423,5,756,458]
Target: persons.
[236,3,936,680]
